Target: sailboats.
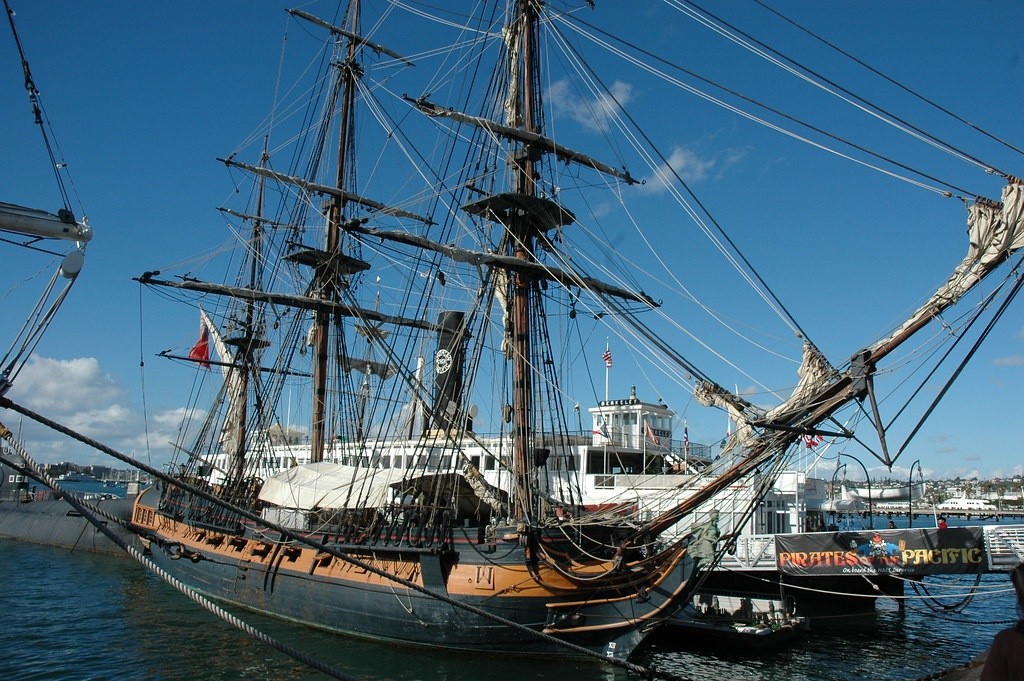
[129,0,1024,666]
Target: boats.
[842,482,926,503]
[0,412,137,555]
[648,570,822,650]
[190,337,904,630]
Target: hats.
[937,517,947,522]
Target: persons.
[937,517,948,528]
[889,521,897,529]
[688,509,720,566]
[980,564,1024,681]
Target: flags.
[684,421,689,451]
[603,349,612,367]
[591,422,610,439]
[644,420,660,447]
[189,315,213,372]
[720,432,729,449]
[802,434,823,448]
